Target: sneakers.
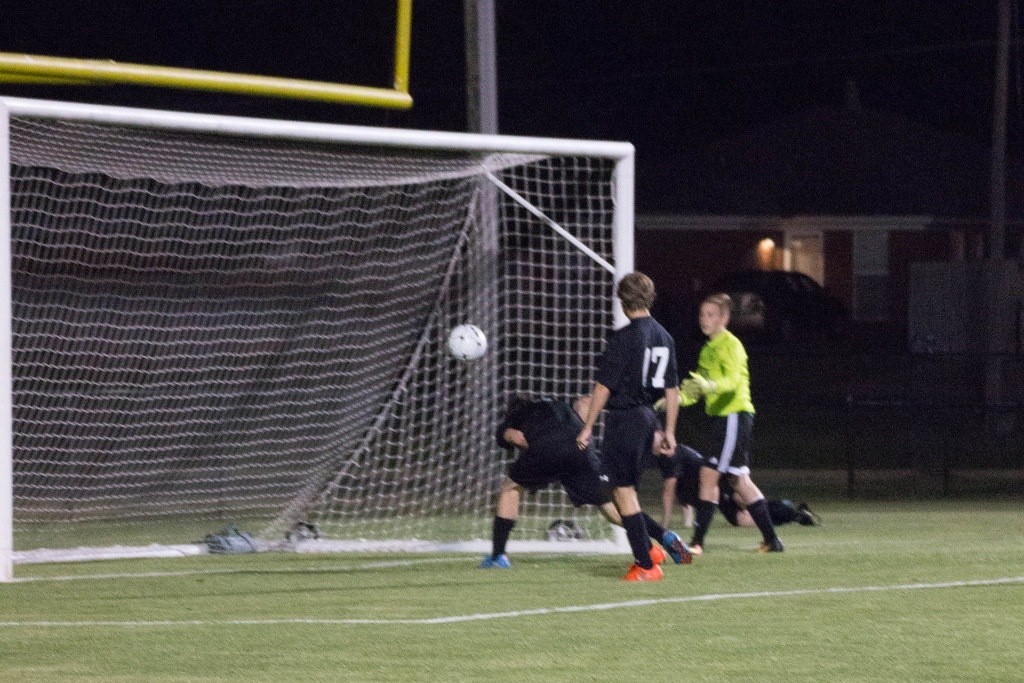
[649,544,666,565]
[758,538,784,552]
[478,553,510,568]
[662,530,693,564]
[623,563,664,580]
[689,543,703,556]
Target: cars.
[654,268,988,443]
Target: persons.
[655,293,784,555]
[577,272,680,581]
[479,397,692,571]
[651,430,826,532]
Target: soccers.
[448,321,489,359]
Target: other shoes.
[798,500,824,526]
[779,499,800,521]
